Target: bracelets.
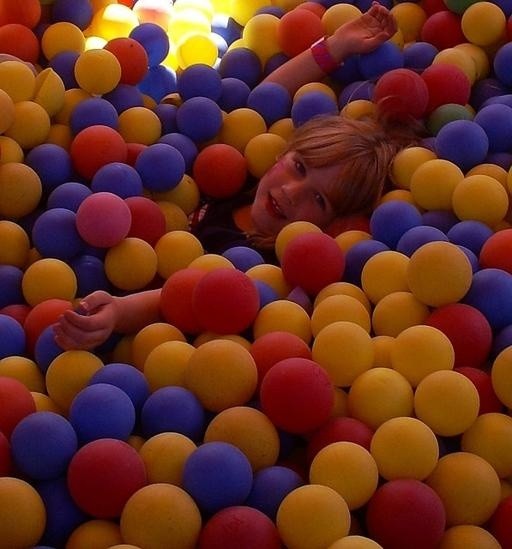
[311,35,345,76]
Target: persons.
[52,1,430,351]
